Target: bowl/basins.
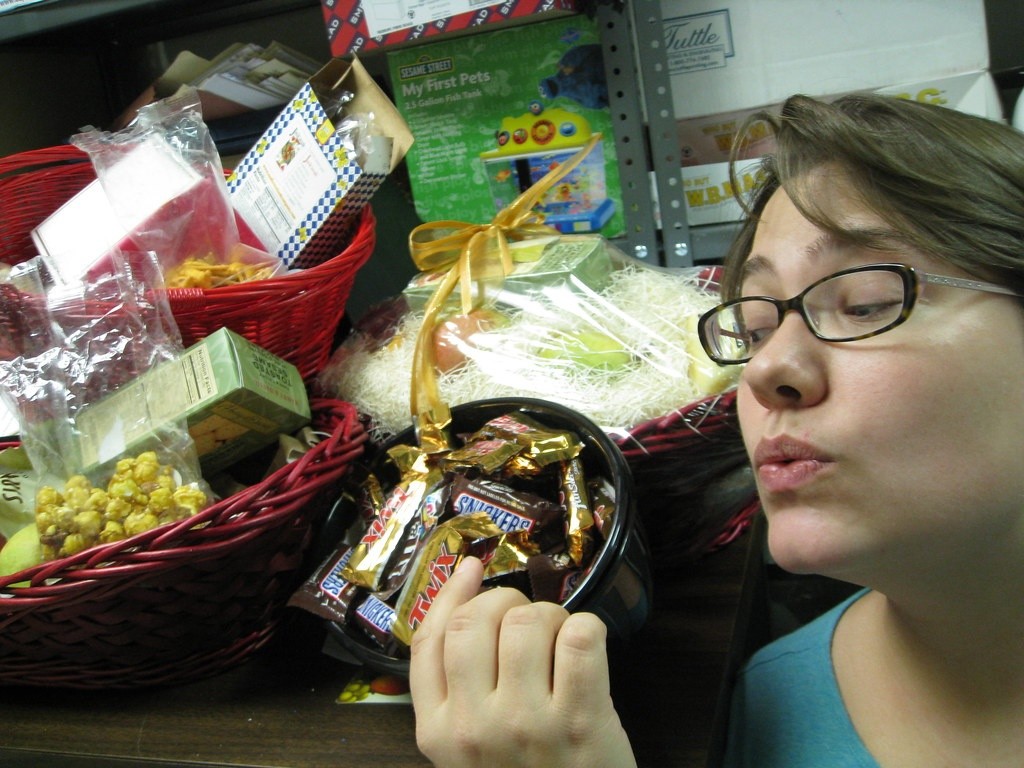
[327,397,660,695]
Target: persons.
[410,91,1024,768]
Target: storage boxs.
[321,0,1004,238]
[30,133,204,287]
[226,49,415,271]
[54,327,310,489]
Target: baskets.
[319,290,765,568]
[0,144,377,424]
[1,401,367,694]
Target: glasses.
[698,265,1020,365]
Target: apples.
[430,309,508,375]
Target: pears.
[537,333,639,373]
[685,309,752,399]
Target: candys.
[286,407,618,657]
